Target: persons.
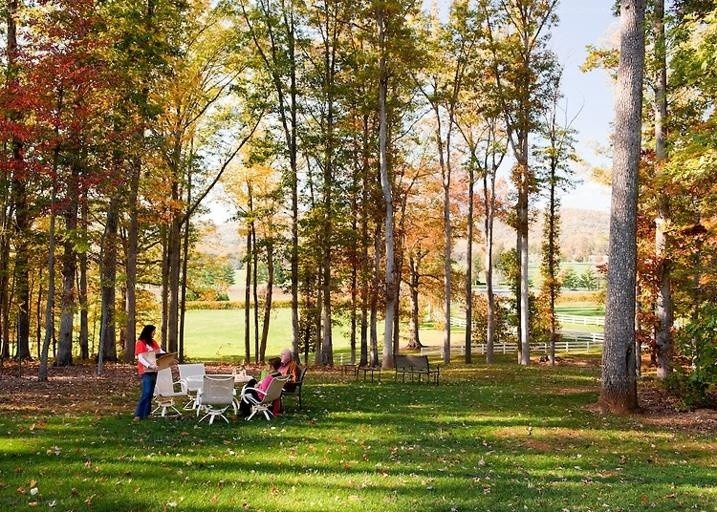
[131,324,180,422]
[264,349,302,418]
[232,357,285,420]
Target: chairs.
[279,368,307,412]
[151,368,187,416]
[199,376,236,424]
[410,354,439,384]
[394,353,423,382]
[239,375,290,420]
[174,364,206,412]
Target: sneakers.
[132,416,140,422]
[147,415,153,419]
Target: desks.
[206,373,253,414]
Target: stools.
[341,365,357,381]
